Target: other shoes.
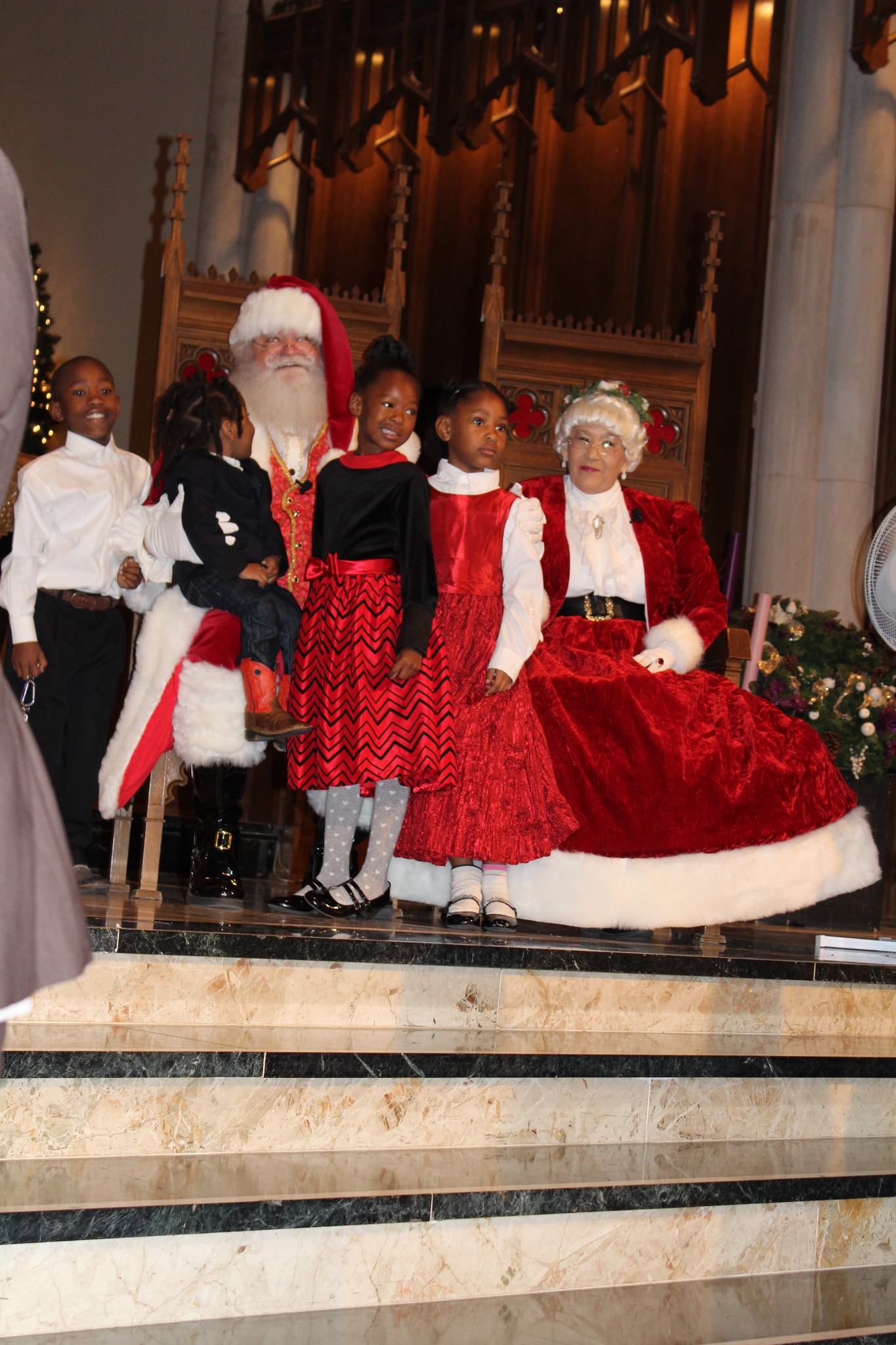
[73,863,107,893]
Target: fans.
[865,504,896,650]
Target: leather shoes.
[444,894,481,931]
[483,897,517,933]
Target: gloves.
[633,642,674,673]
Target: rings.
[656,657,664,666]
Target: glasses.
[567,438,628,458]
[251,337,321,355]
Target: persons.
[267,334,440,919]
[0,355,167,883]
[392,381,581,933]
[107,275,422,909]
[384,378,882,930]
[154,368,313,741]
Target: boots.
[274,674,293,752]
[187,762,246,908]
[240,658,313,742]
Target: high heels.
[268,877,325,916]
[304,878,390,920]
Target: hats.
[228,274,356,473]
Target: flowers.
[728,596,896,783]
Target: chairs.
[108,140,415,900]
[477,182,752,945]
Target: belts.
[40,588,124,611]
[554,593,646,622]
